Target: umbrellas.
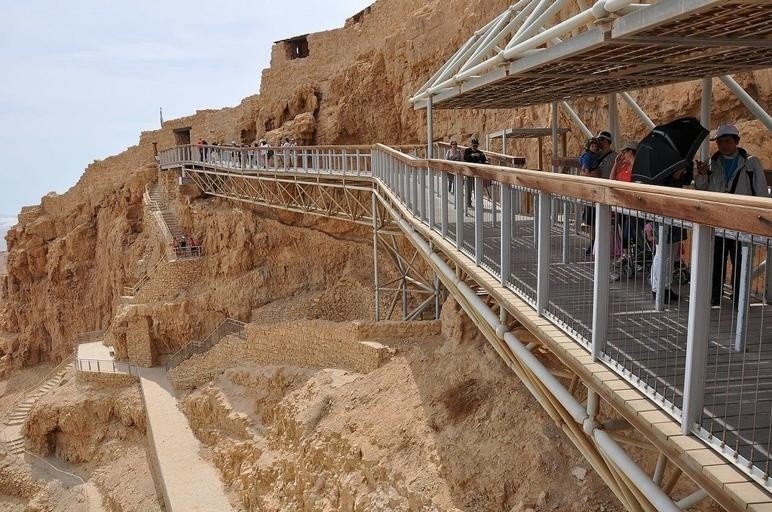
[631,116,711,184]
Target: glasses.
[472,145,478,147]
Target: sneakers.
[586,241,594,255]
[612,254,629,267]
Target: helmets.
[471,139,479,144]
[585,139,599,151]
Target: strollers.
[609,217,689,284]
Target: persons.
[197,136,298,170]
[445,138,486,210]
[578,125,771,310]
[171,236,197,260]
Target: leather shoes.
[712,298,738,311]
[653,290,681,305]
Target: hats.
[616,143,637,152]
[710,124,739,141]
[596,131,614,141]
